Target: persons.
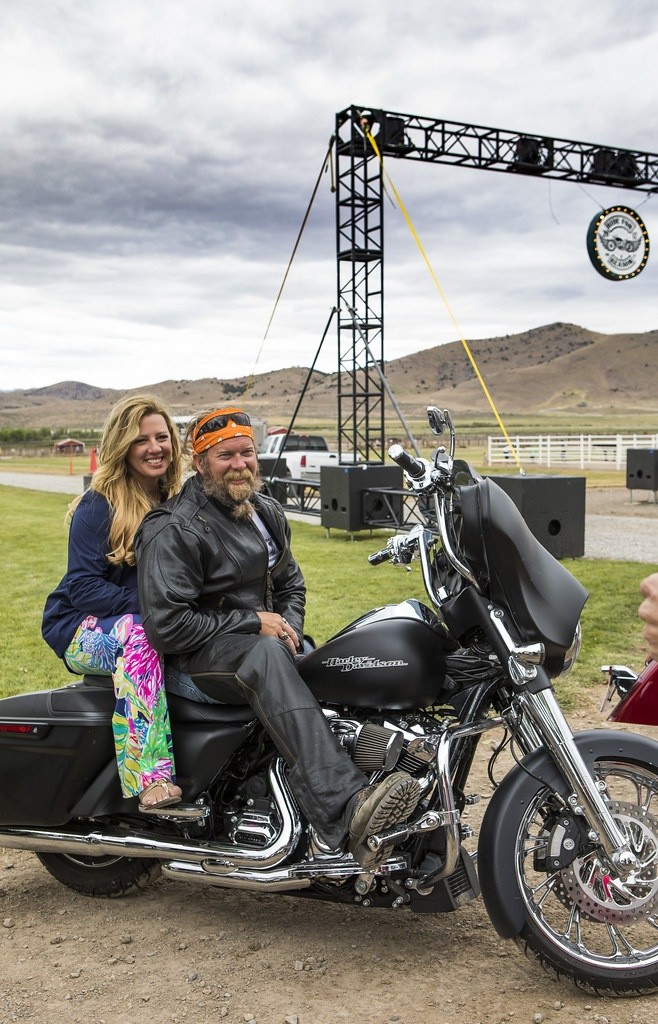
[637,572,658,661]
[134,409,420,869]
[41,393,182,808]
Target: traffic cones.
[86,446,98,476]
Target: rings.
[280,631,289,640]
[283,618,286,623]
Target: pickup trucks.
[255,429,362,498]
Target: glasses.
[192,412,252,449]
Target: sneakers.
[344,771,420,872]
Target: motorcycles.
[0,404,658,1000]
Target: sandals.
[140,779,181,811]
